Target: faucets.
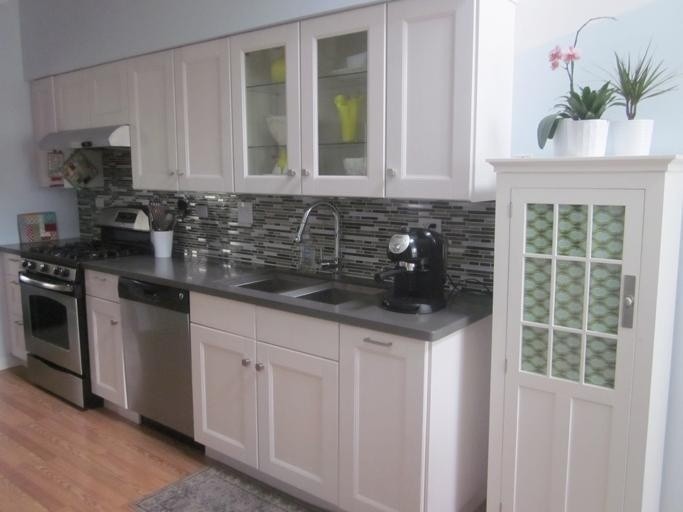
[294,202,343,274]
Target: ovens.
[18,256,83,377]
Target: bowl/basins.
[266,114,287,147]
[343,157,365,175]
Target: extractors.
[37,124,129,149]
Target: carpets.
[128,457,330,512]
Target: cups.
[152,230,174,258]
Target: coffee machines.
[376,227,446,315]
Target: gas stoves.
[19,239,146,264]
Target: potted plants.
[611,51,653,158]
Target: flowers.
[541,44,605,117]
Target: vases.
[556,120,606,157]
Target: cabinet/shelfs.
[29,60,126,188]
[4,254,25,360]
[485,156,680,512]
[338,323,427,512]
[386,1,476,199]
[126,38,235,192]
[228,3,386,198]
[191,290,338,506]
[85,270,127,408]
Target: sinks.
[218,271,328,294]
[278,282,386,307]
